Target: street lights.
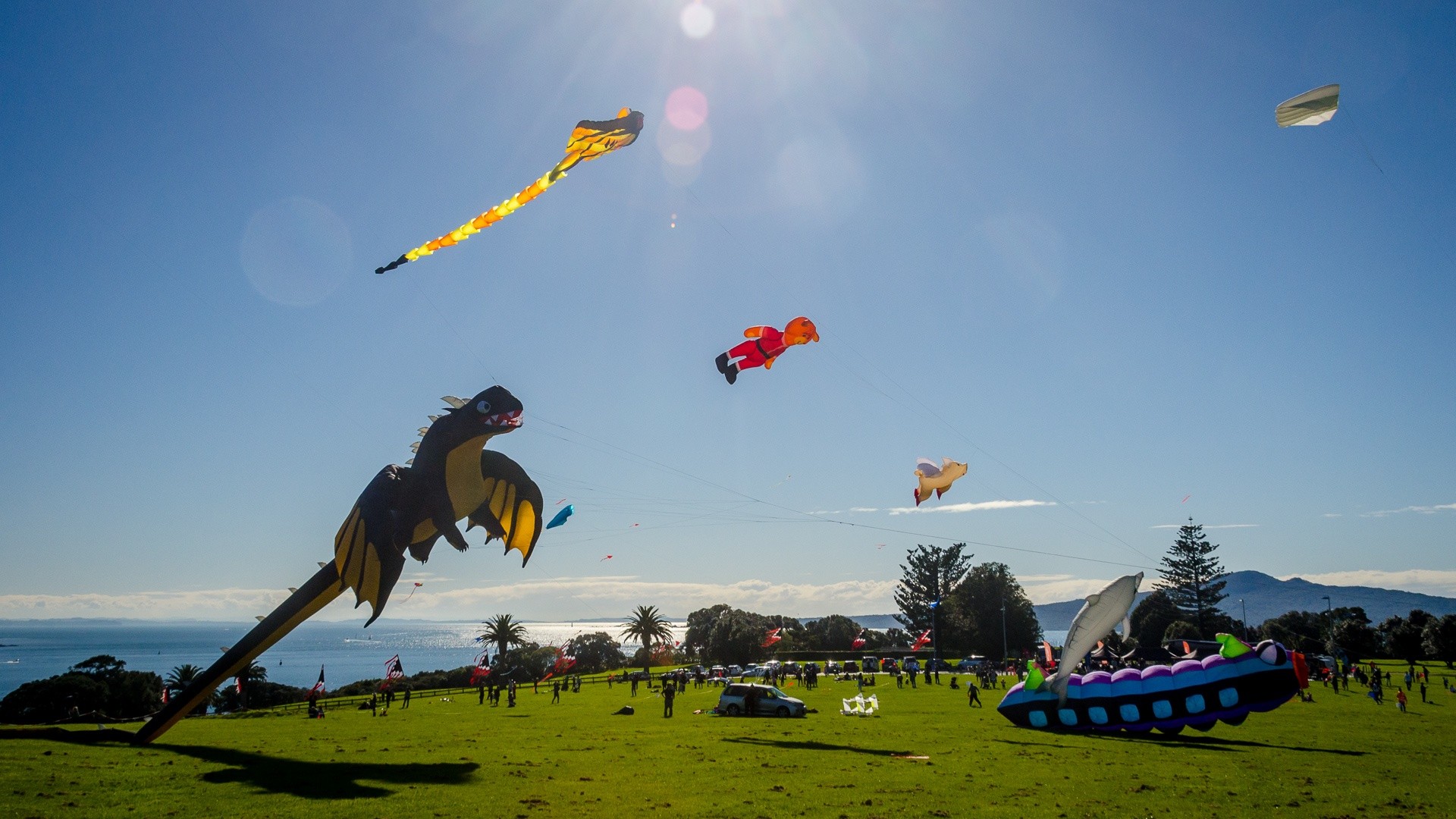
[1239,598,1248,644]
[1321,594,1334,634]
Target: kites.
[600,555,612,563]
[400,583,422,604]
[914,457,967,506]
[374,108,645,274]
[715,317,820,385]
[0,385,544,745]
[1047,572,1144,702]
[1274,84,1339,128]
[998,633,1309,737]
[546,504,573,529]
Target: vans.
[862,656,879,671]
[902,656,920,670]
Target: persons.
[608,671,652,697]
[1443,677,1456,693]
[968,683,982,707]
[889,659,1007,691]
[308,706,325,719]
[1298,687,1311,702]
[358,685,411,717]
[744,685,756,715]
[662,683,675,717]
[662,667,746,696]
[764,658,875,692]
[479,676,582,707]
[1322,661,1430,712]
[1008,651,1146,683]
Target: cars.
[712,665,725,673]
[782,662,799,673]
[620,671,651,681]
[718,682,808,718]
[728,664,742,675]
[824,661,842,673]
[882,658,898,672]
[804,662,821,673]
[746,663,759,671]
[843,661,859,672]
[765,660,781,672]
[742,666,773,677]
[925,658,950,671]
[958,655,987,669]
